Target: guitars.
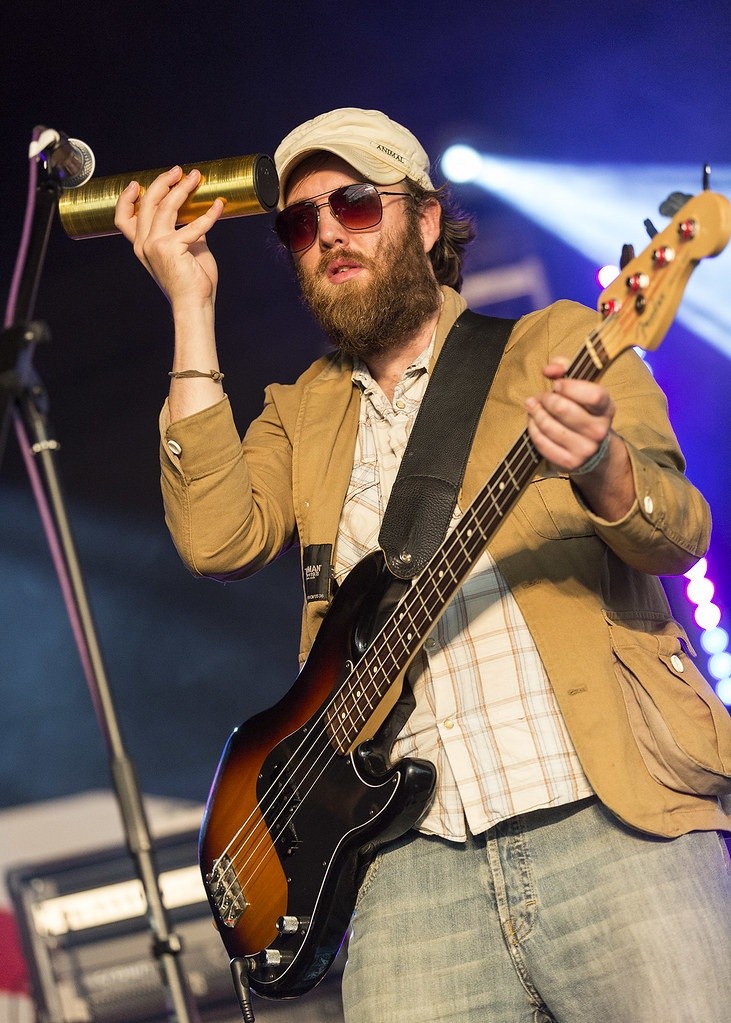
[189,159,731,1003]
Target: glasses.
[270,183,417,253]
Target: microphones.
[33,125,95,190]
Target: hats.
[273,107,436,212]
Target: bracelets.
[168,369,226,382]
[536,431,612,478]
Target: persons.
[110,107,731,1023]
